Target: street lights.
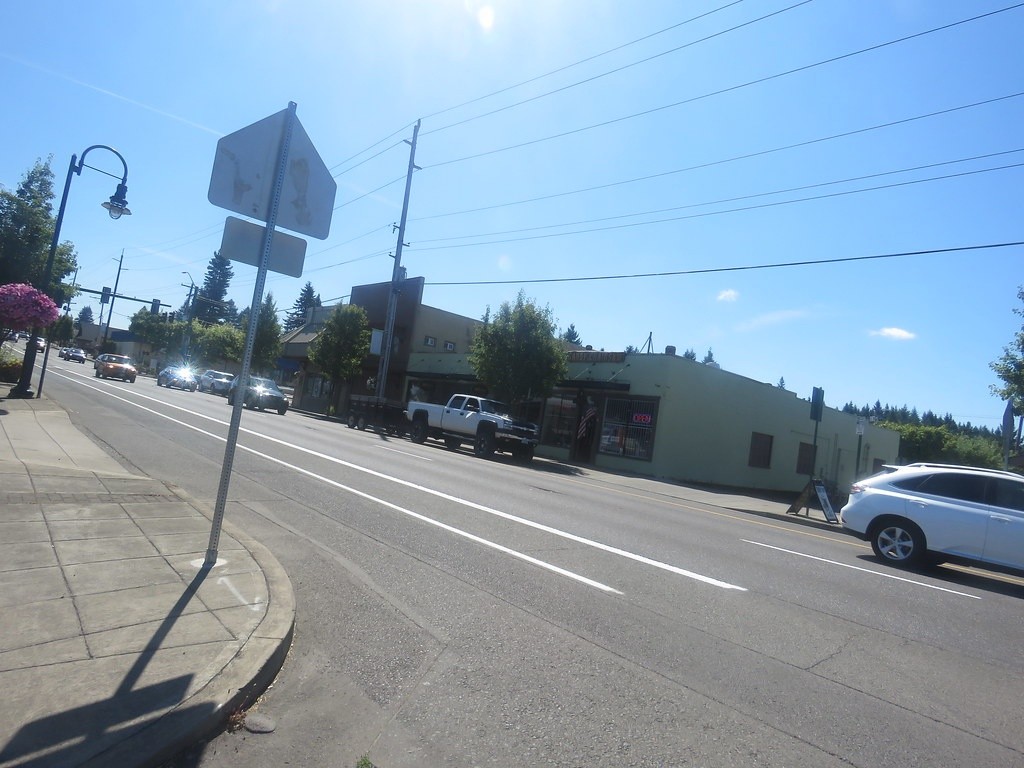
[11,142,133,401]
[182,271,199,357]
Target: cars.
[156,365,197,392]
[8,330,138,384]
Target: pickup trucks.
[404,393,541,464]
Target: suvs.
[198,369,235,396]
[839,461,1024,579]
[226,372,290,416]
